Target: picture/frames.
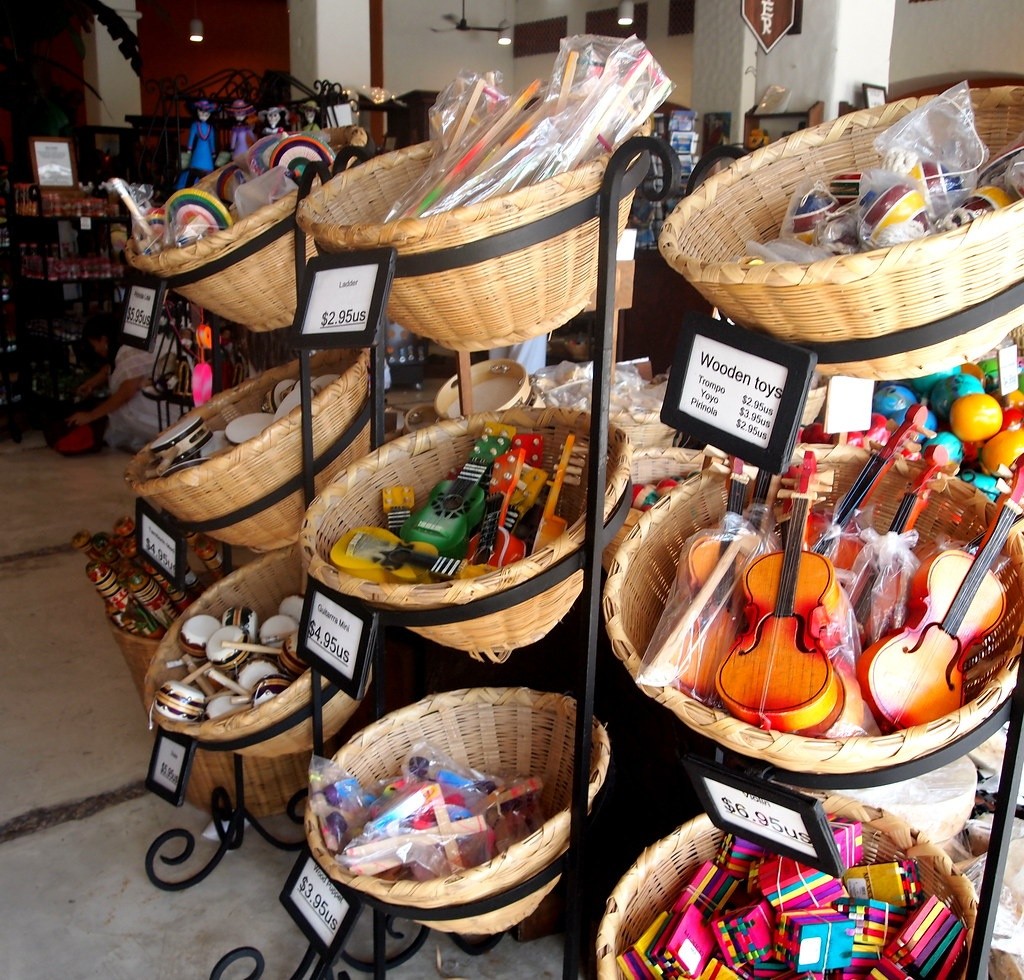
[29,137,78,191]
[862,83,887,109]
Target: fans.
[428,0,503,33]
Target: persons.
[65,312,194,455]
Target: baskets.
[533,376,828,580]
[297,116,651,352]
[303,408,632,663]
[603,443,1024,776]
[304,687,610,935]
[105,546,386,815]
[123,346,371,553]
[597,790,978,980]
[124,125,369,332]
[659,86,1024,379]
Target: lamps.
[188,0,203,42]
[496,0,512,45]
[616,0,633,25]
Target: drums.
[150,371,341,482]
[146,587,310,735]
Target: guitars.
[326,417,586,590]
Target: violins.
[632,404,1024,745]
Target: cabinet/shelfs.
[741,100,825,150]
[0,173,132,443]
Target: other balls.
[737,83,1024,266]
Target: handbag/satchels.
[42,396,108,457]
